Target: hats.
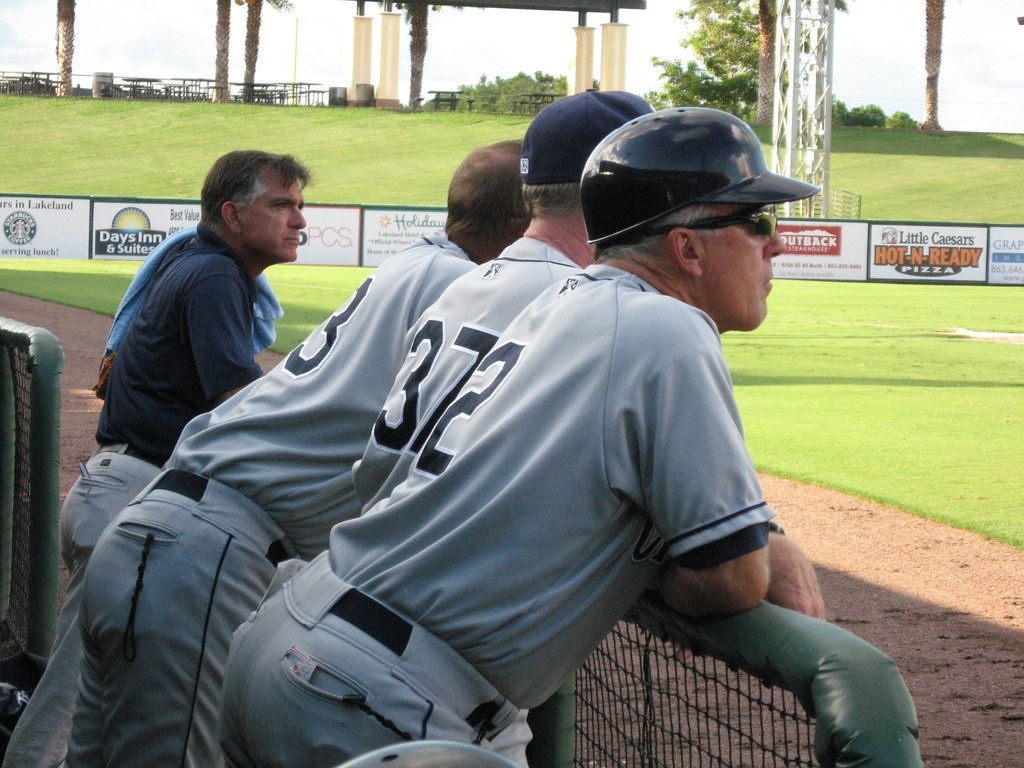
[520,88,654,185]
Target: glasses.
[664,202,778,237]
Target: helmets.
[580,108,820,246]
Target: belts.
[96,442,171,469]
[329,590,517,743]
[152,469,292,566]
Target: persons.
[219,106,826,768]
[355,87,656,513]
[62,138,526,768]
[1,150,308,768]
